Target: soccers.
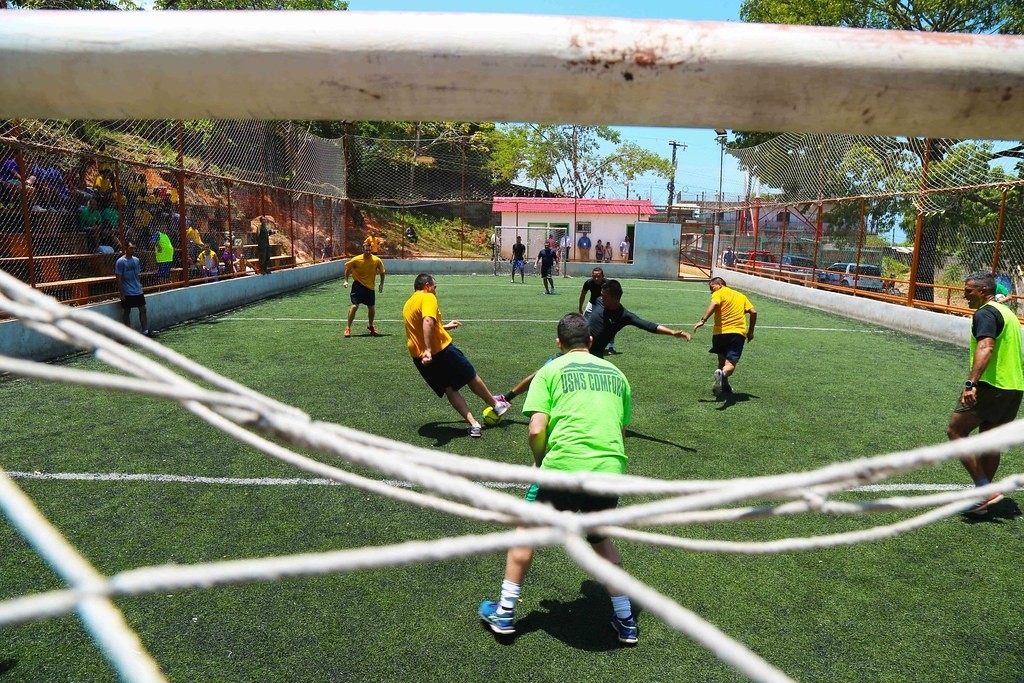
[482,407,503,426]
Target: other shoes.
[31,205,46,213]
[608,349,617,354]
[48,206,69,213]
[0,202,16,210]
[142,330,156,338]
[510,279,514,283]
[521,281,524,283]
[261,269,271,275]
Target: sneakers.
[712,369,732,399]
[478,601,516,635]
[960,492,1004,515]
[545,290,555,294]
[344,329,351,336]
[367,324,377,334]
[611,613,639,643]
[493,400,512,416]
[492,395,506,402]
[466,426,482,436]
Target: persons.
[946,272,1024,514]
[115,241,157,338]
[402,273,512,438]
[364,231,381,255]
[510,236,527,283]
[321,237,332,262]
[405,223,418,246]
[491,230,500,261]
[882,273,897,304]
[534,243,560,295]
[1,141,279,283]
[579,267,616,355]
[620,235,630,264]
[546,232,612,263]
[343,243,385,336]
[491,279,691,403]
[723,246,738,270]
[694,277,757,398]
[477,312,639,646]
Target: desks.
[734,263,817,289]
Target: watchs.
[701,317,707,323]
[965,380,979,389]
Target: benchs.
[0,202,296,316]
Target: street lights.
[715,126,727,224]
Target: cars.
[818,262,885,296]
[680,246,776,277]
[762,255,817,285]
